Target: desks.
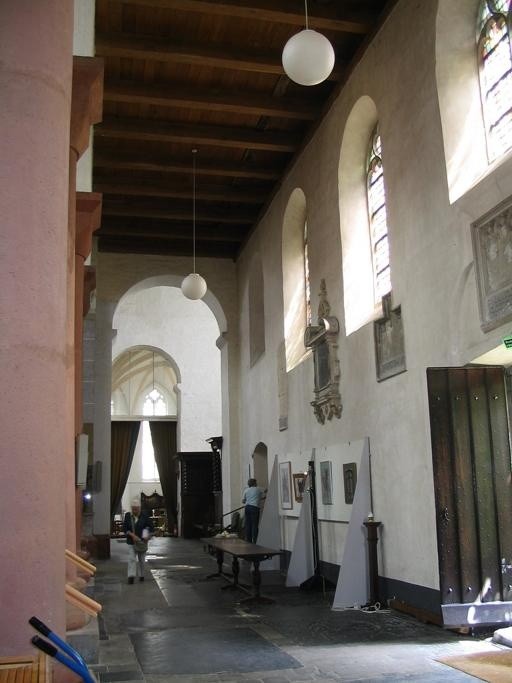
[199,537,285,606]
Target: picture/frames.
[293,473,306,503]
[461,194,512,334]
[320,461,333,505]
[343,463,357,504]
[279,462,293,510]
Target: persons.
[242,478,267,544]
[123,500,150,584]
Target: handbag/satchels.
[134,540,148,553]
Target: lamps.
[282,0,336,87]
[181,149,207,300]
